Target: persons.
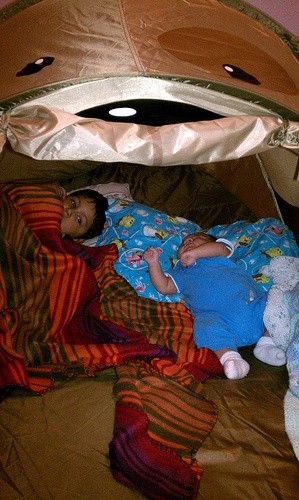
[143,232,287,379]
[61,189,109,244]
